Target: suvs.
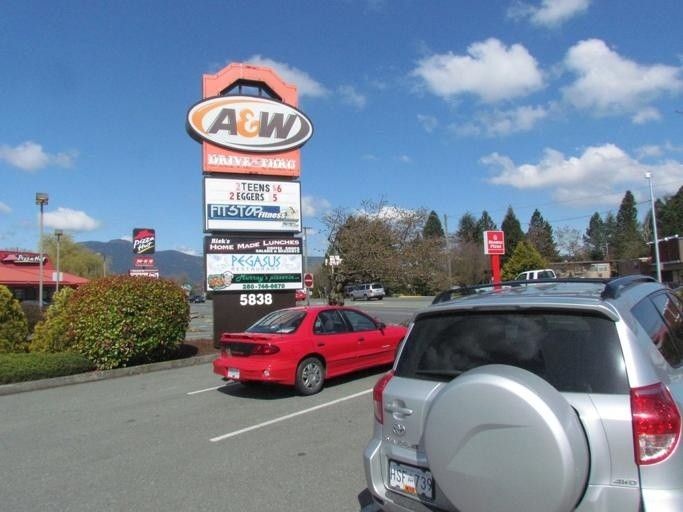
[363,275,683,512]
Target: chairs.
[321,316,338,334]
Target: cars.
[515,269,557,281]
[295,291,305,300]
[213,305,410,396]
[190,295,206,303]
[350,283,386,301]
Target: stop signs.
[304,273,314,288]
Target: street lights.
[36,192,49,310]
[55,229,63,293]
[645,170,662,282]
